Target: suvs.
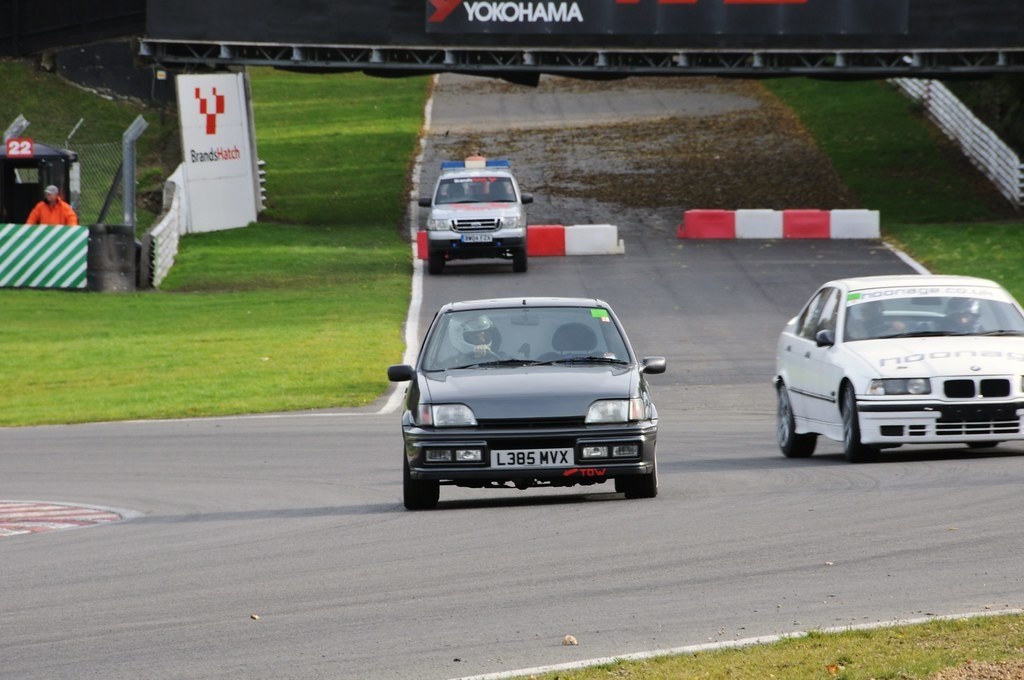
[427,153,534,274]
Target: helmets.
[448,314,493,358]
[944,296,979,316]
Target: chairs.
[441,327,508,369]
[535,322,607,361]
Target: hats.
[44,184,59,194]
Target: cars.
[386,298,663,509]
[770,270,1023,465]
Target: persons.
[440,314,509,367]
[24,185,79,225]
[489,182,508,199]
[448,183,465,199]
[943,298,985,334]
[850,306,904,340]
